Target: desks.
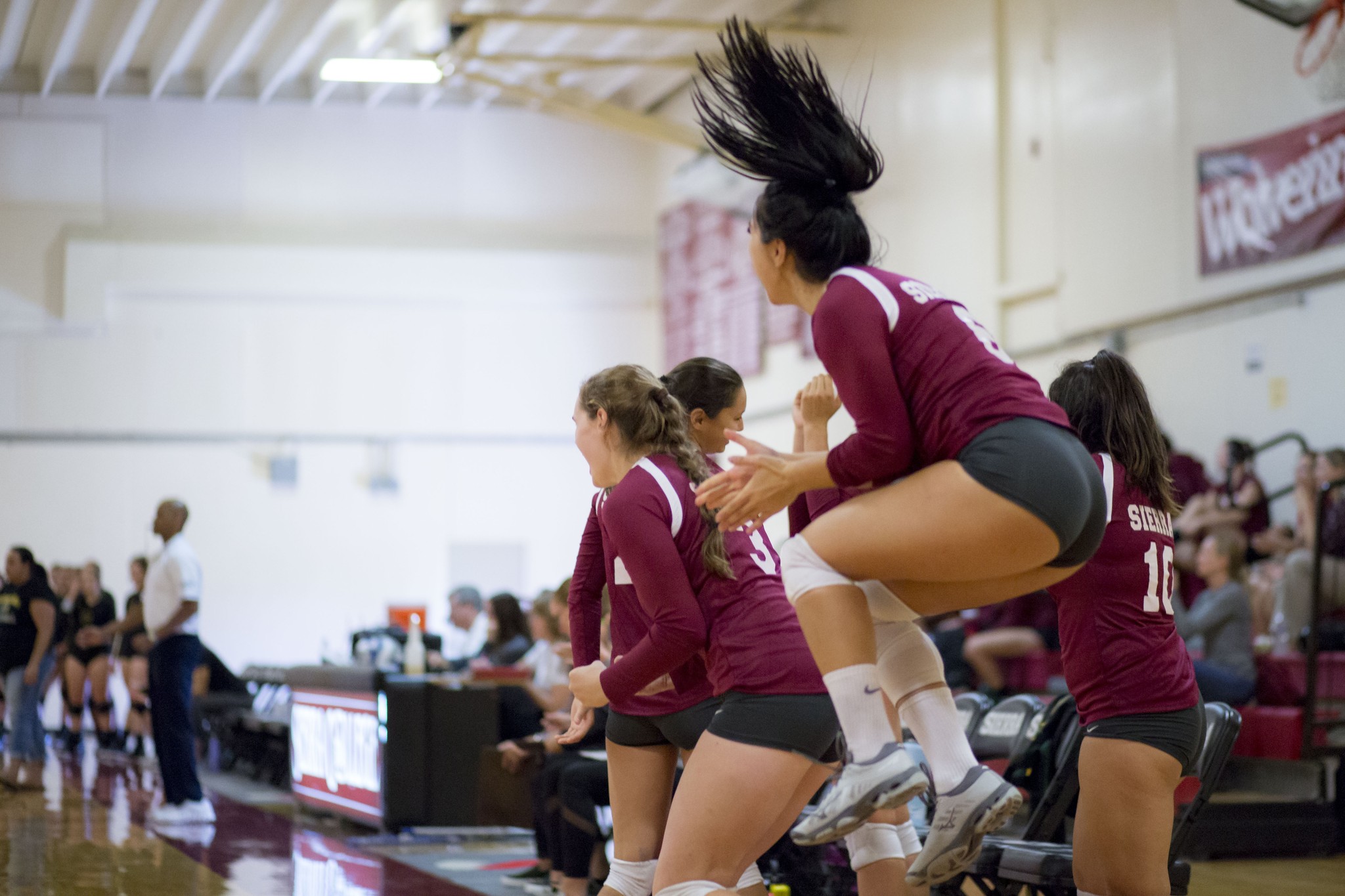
[287,667,544,835]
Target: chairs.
[193,666,293,784]
[579,689,1242,896]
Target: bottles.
[405,620,425,676]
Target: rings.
[757,513,765,521]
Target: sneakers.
[904,763,1024,887]
[790,730,931,847]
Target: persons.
[140,500,216,826]
[0,548,253,760]
[435,12,1345,896]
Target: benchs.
[980,624,1345,808]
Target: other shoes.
[149,798,218,823]
[523,882,558,896]
[500,866,549,885]
